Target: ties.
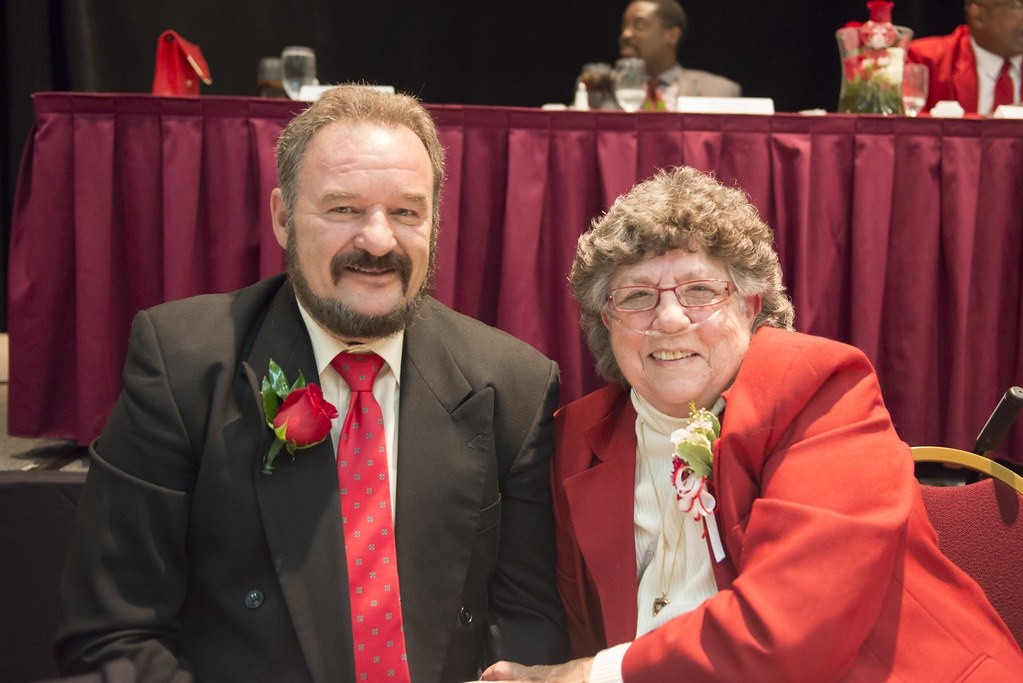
[643,79,663,112]
[992,59,1014,113]
[321,350,408,683]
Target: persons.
[575,0,742,98]
[478,167,1023,683]
[901,0,1023,118]
[50,85,570,683]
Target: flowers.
[670,400,721,521]
[260,357,339,476]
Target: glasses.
[605,280,738,312]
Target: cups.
[614,59,648,111]
[281,48,315,101]
[903,65,928,118]
[581,64,611,110]
[258,59,284,97]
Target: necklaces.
[638,422,690,616]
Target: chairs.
[909,446,1023,651]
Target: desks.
[9,92,1023,467]
[0,437,93,683]
